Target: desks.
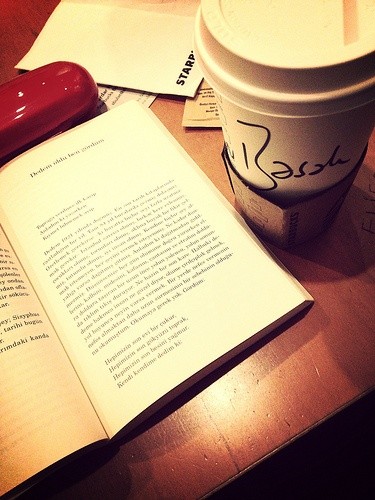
[0,0,375,499]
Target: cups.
[193,0,375,203]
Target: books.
[0,98,315,500]
